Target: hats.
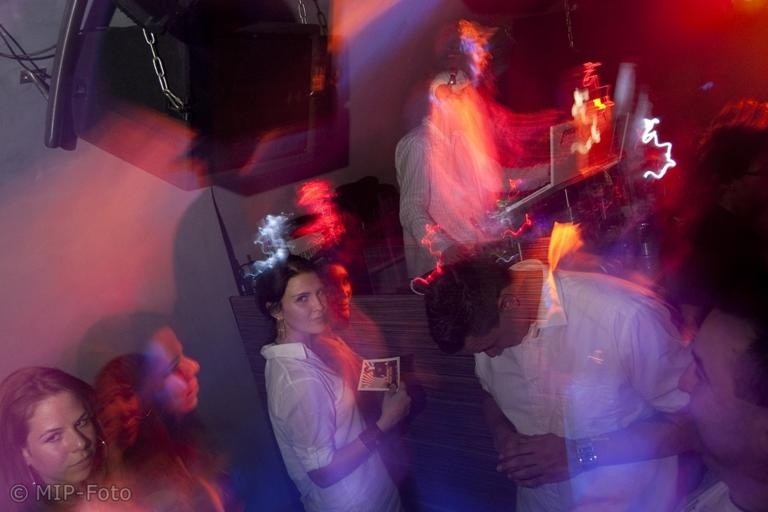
[428,68,479,103]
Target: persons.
[0,13,768,512]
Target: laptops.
[504,109,617,213]
